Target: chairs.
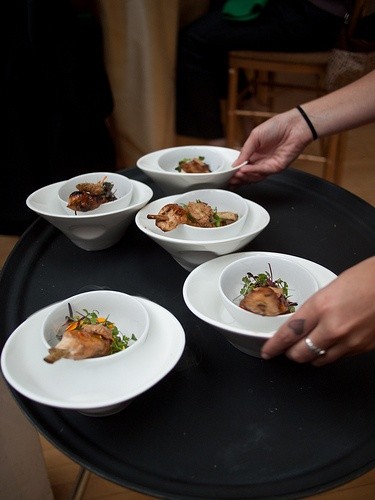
[226,0,368,188]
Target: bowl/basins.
[137,146,251,194]
[135,195,269,270]
[157,147,225,173]
[219,255,321,334]
[175,188,247,237]
[181,250,337,359]
[3,295,186,417]
[26,178,153,252]
[59,171,133,216]
[42,290,148,370]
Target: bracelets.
[295,105,317,141]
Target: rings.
[304,337,325,357]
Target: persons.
[230,69,374,366]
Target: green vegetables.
[62,302,137,355]
[101,181,113,192]
[179,200,227,227]
[175,155,205,172]
[232,263,298,313]
